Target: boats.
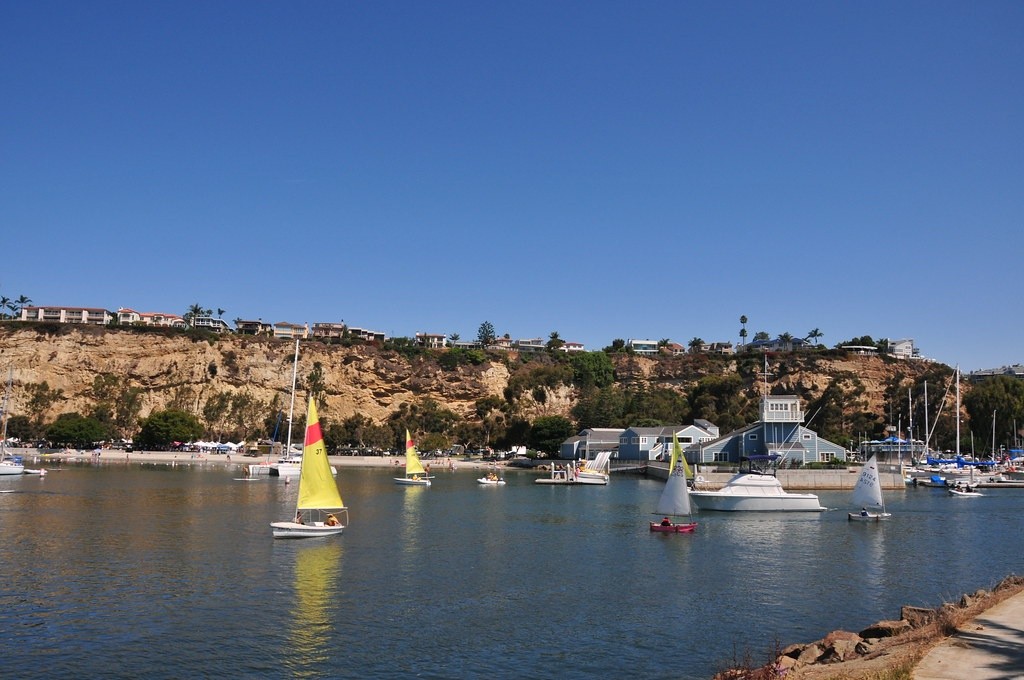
[24,467,48,474]
[902,371,1024,499]
[688,452,827,514]
[477,477,505,485]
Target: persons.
[555,460,583,483]
[660,516,671,528]
[953,483,977,495]
[292,511,304,527]
[324,514,339,528]
[479,471,500,482]
[8,435,246,467]
[243,466,250,480]
[410,463,430,482]
[860,508,869,516]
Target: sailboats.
[647,454,697,534]
[0,365,23,476]
[270,393,348,538]
[846,448,892,522]
[246,338,336,476]
[392,429,432,485]
